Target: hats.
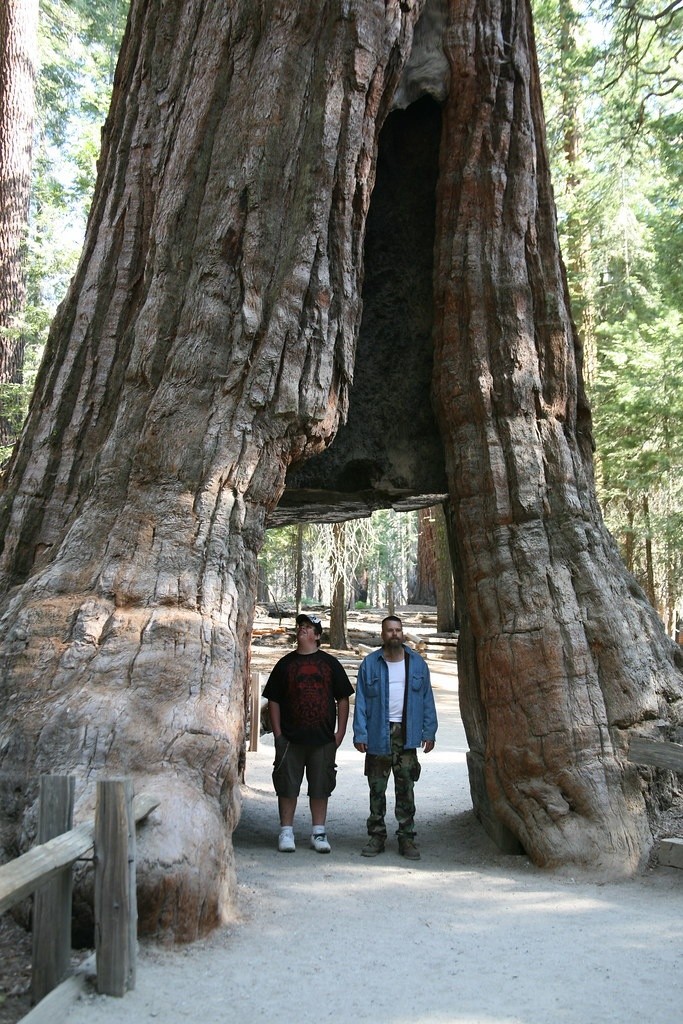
[296,613,322,632]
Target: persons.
[261,613,356,852]
[352,615,438,859]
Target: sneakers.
[397,836,421,860]
[361,834,386,856]
[278,829,297,852]
[310,832,331,853]
